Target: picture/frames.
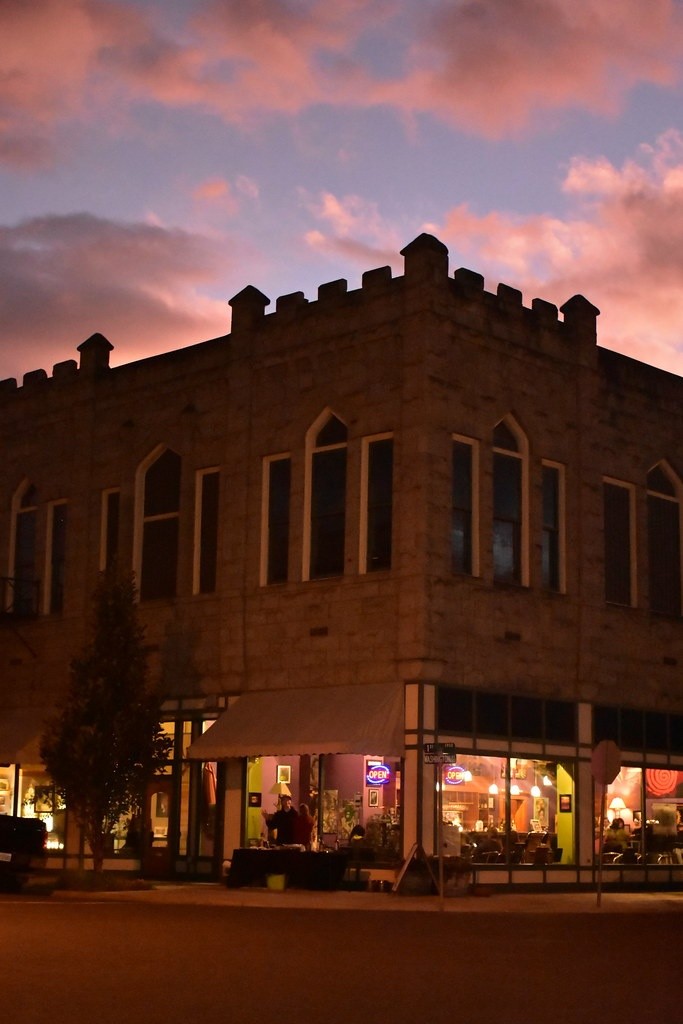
[277,765,291,784]
[366,759,382,787]
[368,789,379,807]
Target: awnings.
[185,681,405,761]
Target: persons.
[260,795,297,843]
[291,803,315,849]
[595,812,683,859]
[449,819,524,864]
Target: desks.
[225,848,353,890]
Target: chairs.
[460,831,683,865]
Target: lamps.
[609,797,626,819]
[269,782,291,811]
